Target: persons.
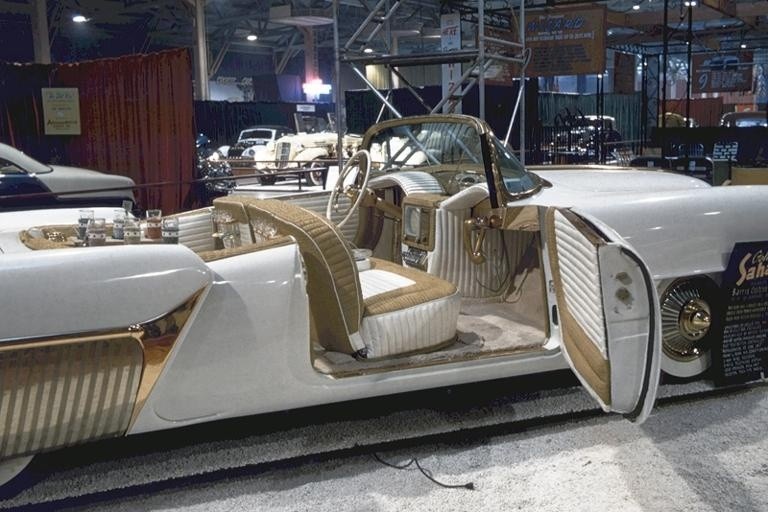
[603,119,621,141]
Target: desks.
[0,208,211,342]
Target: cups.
[209,210,279,250]
[74,199,180,245]
